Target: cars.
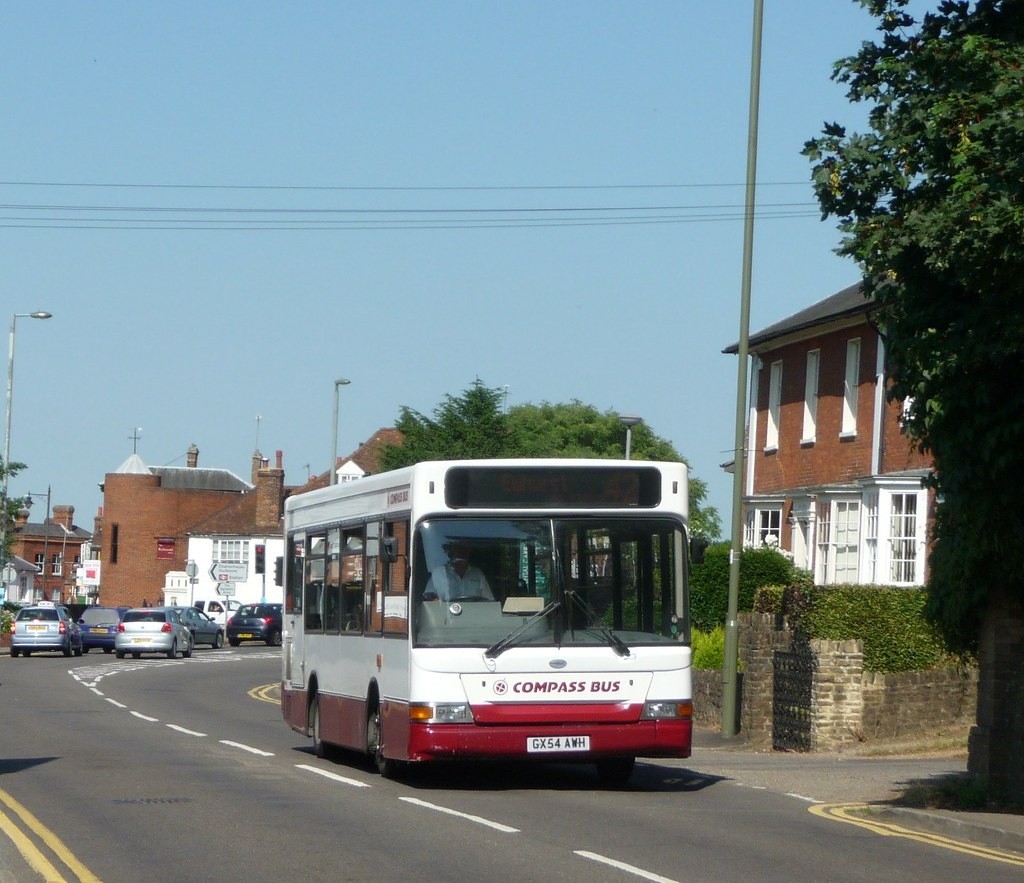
[173,606,225,650]
[116,607,194,658]
[78,604,132,654]
[226,603,283,647]
[10,601,84,658]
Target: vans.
[193,598,247,636]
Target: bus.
[280,459,692,783]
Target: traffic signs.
[215,583,235,597]
[209,563,248,583]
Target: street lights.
[1,312,52,591]
[328,379,351,486]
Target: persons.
[422,544,495,602]
